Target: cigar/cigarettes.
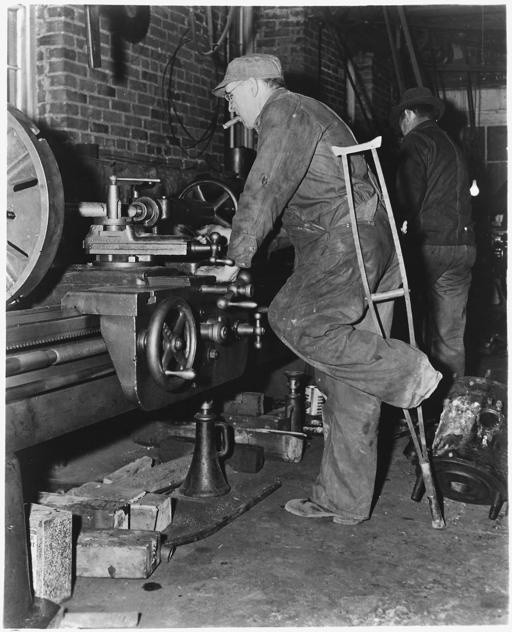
[222,115,241,129]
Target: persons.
[195,53,443,524]
[387,87,478,386]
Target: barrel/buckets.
[410,374,507,518]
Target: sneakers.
[284,499,360,527]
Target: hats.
[211,55,282,97]
[389,87,446,135]
